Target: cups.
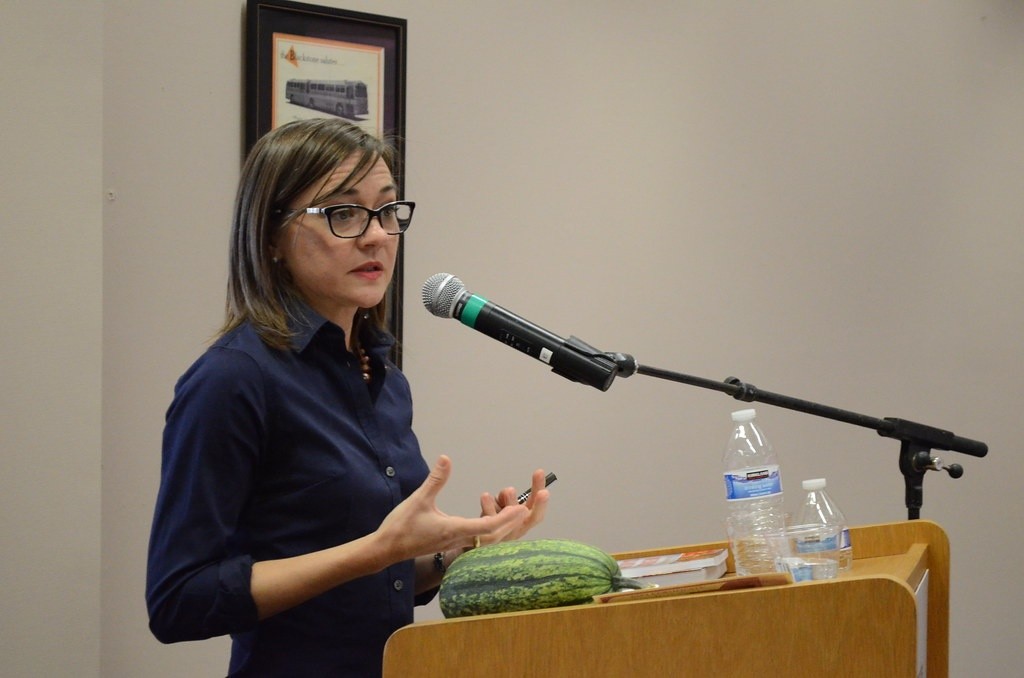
[773,524,842,583]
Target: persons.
[144,118,551,678]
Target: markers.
[517,472,558,510]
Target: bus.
[286,77,368,117]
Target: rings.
[472,534,481,550]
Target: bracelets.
[433,552,448,578]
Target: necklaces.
[358,344,371,382]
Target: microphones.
[421,274,617,392]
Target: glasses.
[273,200,415,240]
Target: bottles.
[794,478,852,572]
[721,408,790,578]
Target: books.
[615,549,732,591]
